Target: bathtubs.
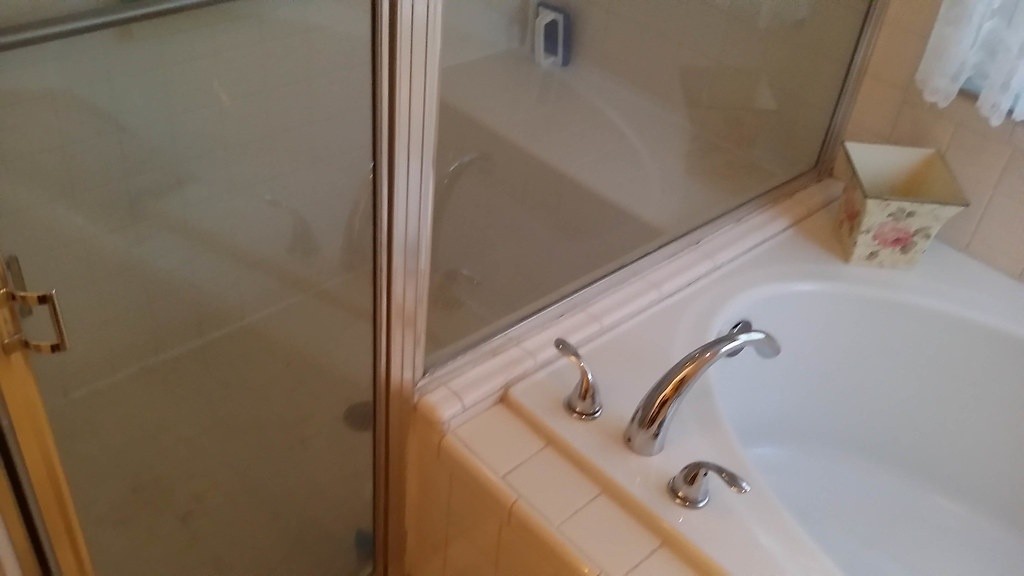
[500,197,1024,575]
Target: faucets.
[620,327,782,459]
